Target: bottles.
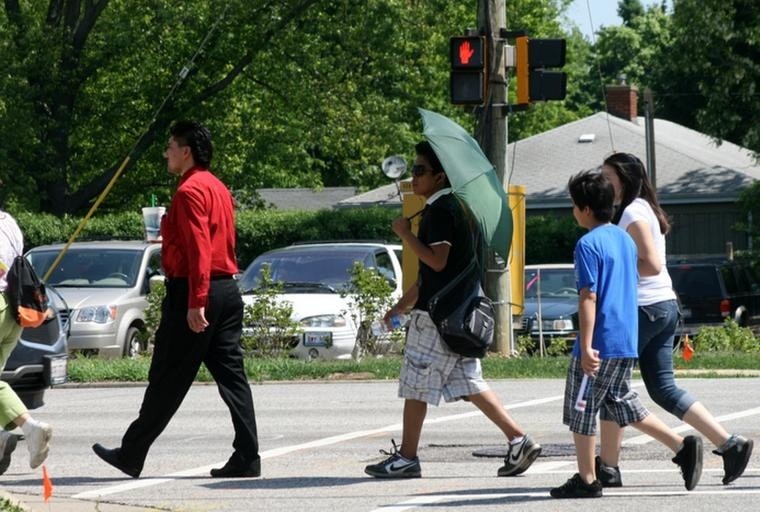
[371,312,412,337]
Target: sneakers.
[26,422,52,469]
[671,435,704,490]
[211,452,260,478]
[595,455,622,487]
[550,474,602,498]
[711,436,753,484]
[0,432,17,478]
[497,433,542,476]
[364,438,422,479]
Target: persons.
[545,172,704,500]
[363,140,543,478]
[90,119,262,479]
[593,152,755,487]
[0,203,54,478]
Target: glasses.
[410,163,429,177]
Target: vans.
[237,242,410,363]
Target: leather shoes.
[91,442,144,479]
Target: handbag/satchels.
[427,256,496,360]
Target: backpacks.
[1,229,49,329]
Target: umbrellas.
[405,104,516,268]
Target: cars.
[511,263,583,355]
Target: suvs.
[22,241,164,360]
[668,261,759,346]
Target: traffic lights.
[451,36,484,104]
[527,39,567,100]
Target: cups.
[141,206,167,244]
